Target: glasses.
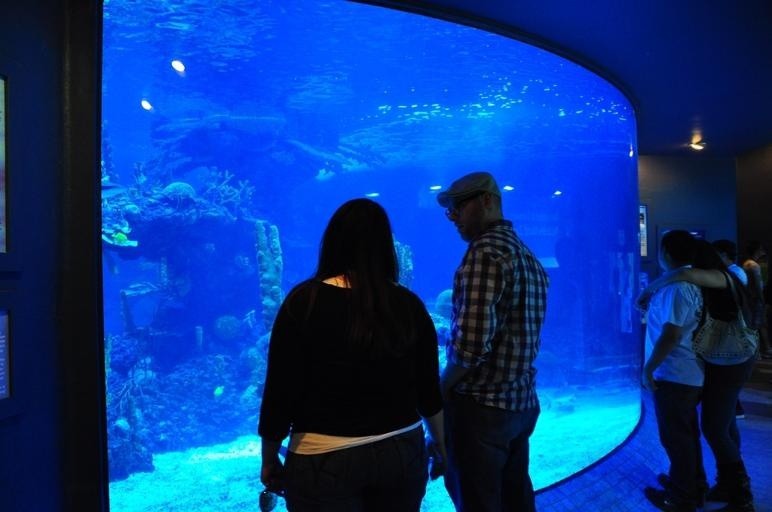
[262,467,275,512]
[445,197,476,217]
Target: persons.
[643,227,712,511]
[711,242,752,417]
[635,237,762,511]
[744,238,770,361]
[429,169,549,511]
[256,199,448,512]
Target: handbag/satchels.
[692,268,761,366]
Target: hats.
[437,172,501,206]
